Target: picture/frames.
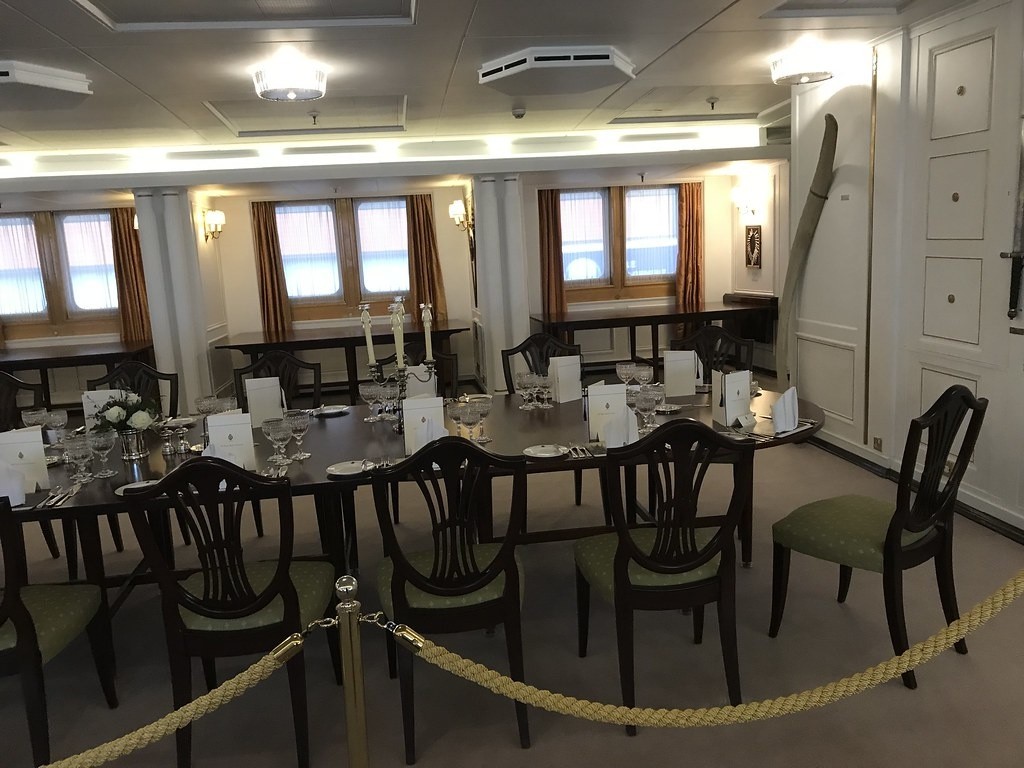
[745,225,761,270]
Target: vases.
[116,426,150,462]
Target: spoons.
[37,485,63,508]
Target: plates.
[163,419,197,428]
[326,460,375,475]
[523,445,568,458]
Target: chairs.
[0,496,118,767]
[0,325,755,560]
[765,383,990,690]
[572,419,756,742]
[122,456,346,766]
[370,434,533,764]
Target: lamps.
[770,54,836,86]
[448,198,476,231]
[202,209,228,242]
[252,63,327,104]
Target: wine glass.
[20,408,118,484]
[194,363,664,465]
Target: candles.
[393,303,405,368]
[361,307,376,365]
[422,303,434,360]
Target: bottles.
[175,425,191,453]
[160,428,177,456]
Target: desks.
[528,300,771,383]
[213,317,470,406]
[0,337,149,413]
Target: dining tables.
[0,381,827,708]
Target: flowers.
[85,382,165,433]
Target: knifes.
[46,483,81,506]
